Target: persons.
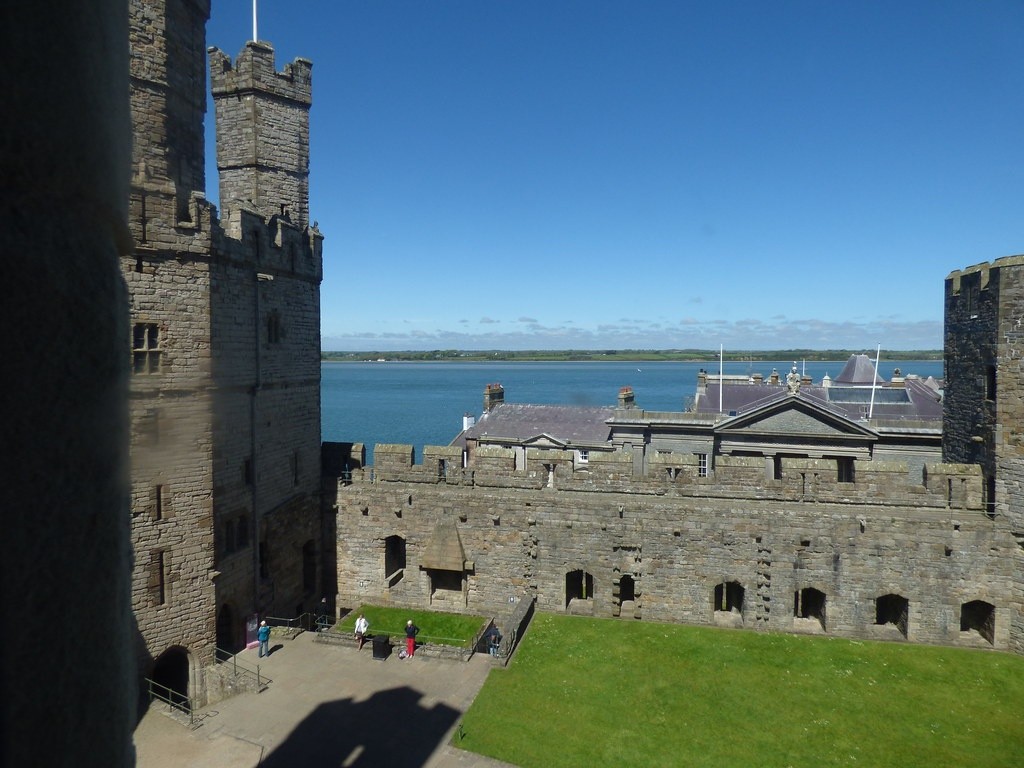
[354,614,370,652]
[256,621,271,658]
[785,367,801,395]
[404,619,420,659]
[486,624,500,656]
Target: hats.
[407,620,412,624]
[261,621,266,626]
